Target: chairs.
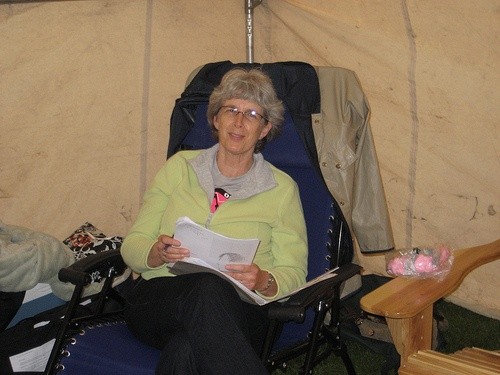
[358,237,500,375]
[43,60,360,375]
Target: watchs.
[259,270,274,292]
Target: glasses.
[218,106,269,122]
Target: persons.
[120,70,308,375]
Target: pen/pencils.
[162,233,175,252]
